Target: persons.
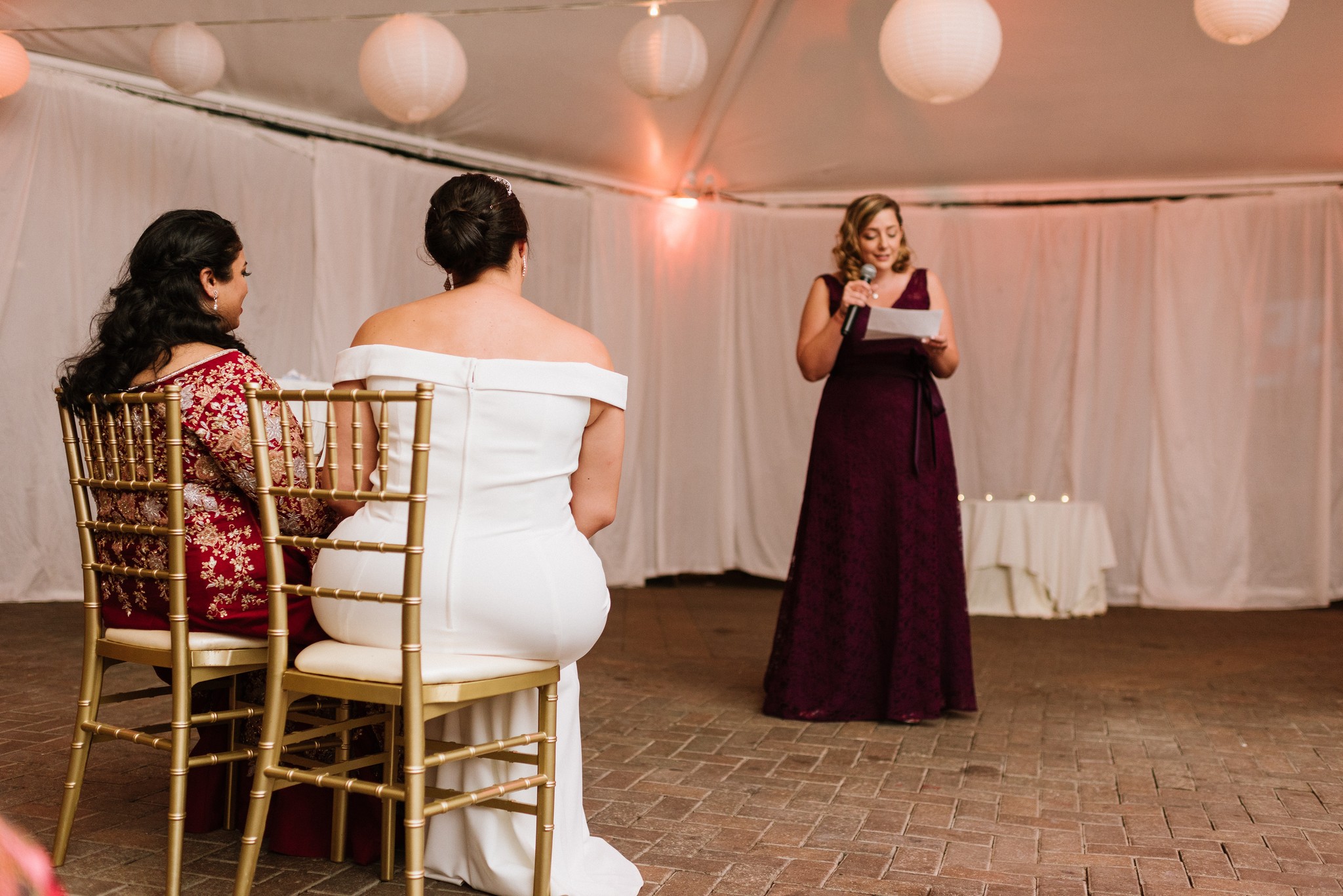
[304,164,651,896]
[761,194,984,726]
[59,208,351,859]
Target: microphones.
[839,261,877,336]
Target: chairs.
[233,381,558,896]
[50,386,354,895]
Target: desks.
[959,492,1116,615]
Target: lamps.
[658,191,695,239]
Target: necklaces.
[869,279,887,300]
